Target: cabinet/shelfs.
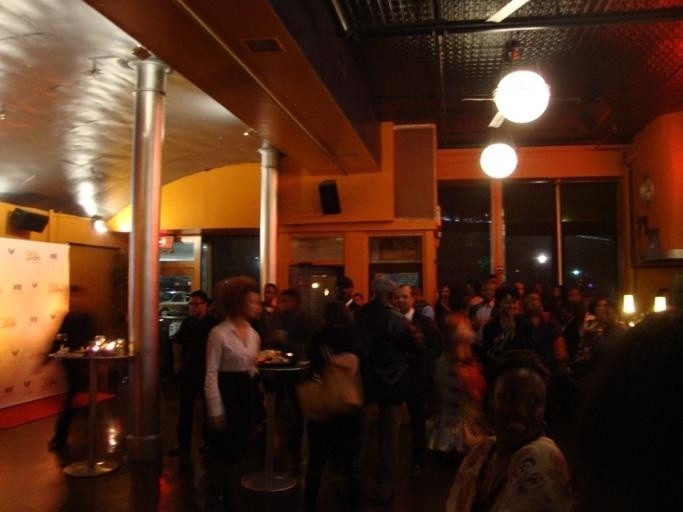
[277,229,436,313]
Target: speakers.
[10,208,50,233]
[318,180,341,215]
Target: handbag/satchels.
[294,346,364,419]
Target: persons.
[33,283,99,449]
[165,268,682,511]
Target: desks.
[49,346,139,478]
[232,357,312,493]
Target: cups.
[56,333,69,348]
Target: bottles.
[114,336,126,355]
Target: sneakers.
[48,433,68,448]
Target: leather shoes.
[168,442,192,456]
[197,440,210,454]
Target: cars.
[158,290,190,318]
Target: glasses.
[186,300,208,305]
